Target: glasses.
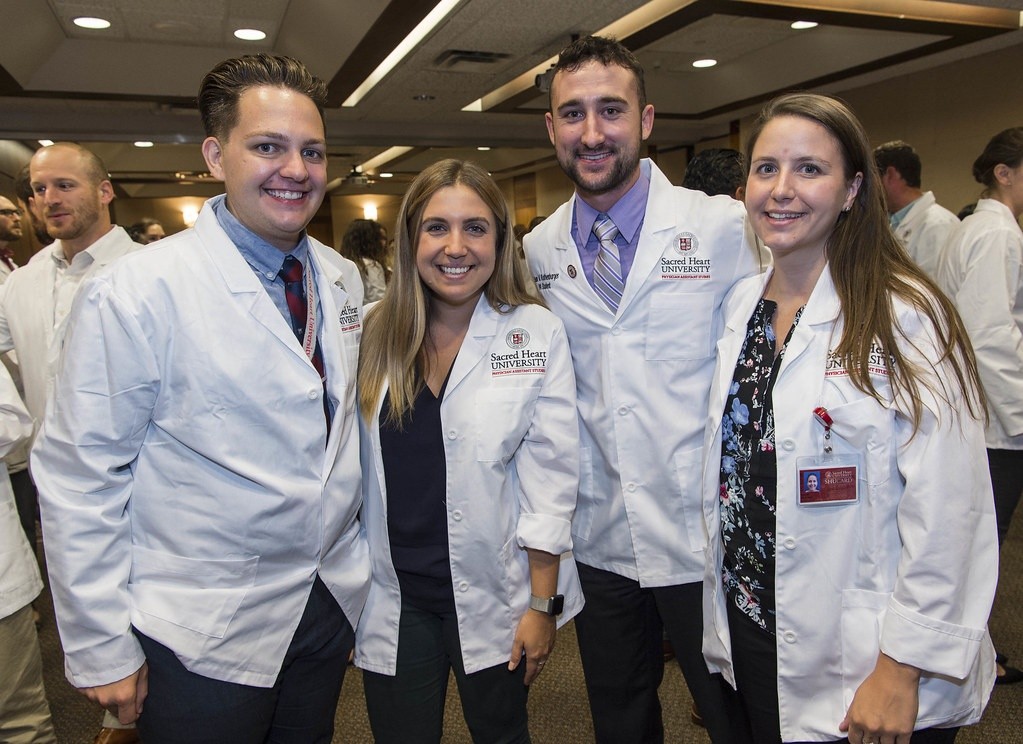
[1,209,24,220]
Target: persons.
[804,472,820,493]
[700,92,998,743]
[0,193,49,629]
[128,218,167,247]
[29,50,372,743]
[867,139,961,287]
[0,141,147,478]
[940,125,1022,553]
[520,30,775,743]
[355,155,583,743]
[337,219,388,305]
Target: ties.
[277,258,332,451]
[588,218,625,317]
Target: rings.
[538,661,546,665]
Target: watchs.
[528,593,564,615]
